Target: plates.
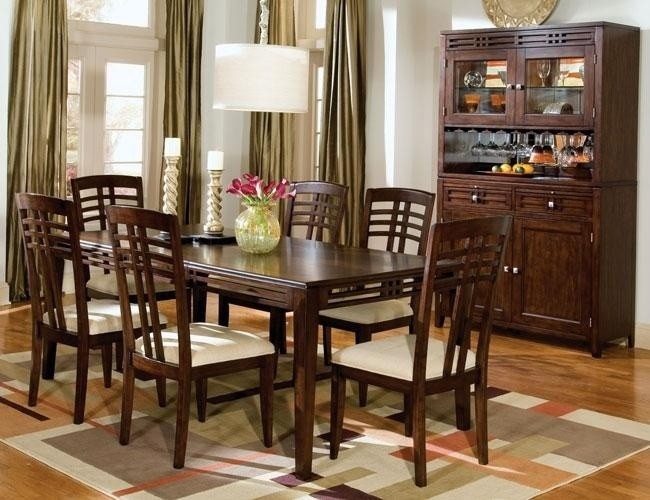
[543,103,573,114]
[480,172,545,178]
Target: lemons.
[522,164,534,174]
[513,164,522,173]
[500,163,511,172]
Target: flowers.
[223,172,298,207]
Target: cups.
[578,66,584,86]
[445,128,594,168]
[490,95,505,113]
[465,94,480,112]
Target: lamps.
[211,1,313,116]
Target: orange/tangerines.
[516,167,524,173]
[492,165,501,173]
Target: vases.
[233,201,281,255]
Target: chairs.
[105,202,279,471]
[326,213,515,488]
[218,180,349,355]
[14,189,171,425]
[318,185,440,407]
[68,173,192,372]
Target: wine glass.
[556,58,569,86]
[536,59,550,87]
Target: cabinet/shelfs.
[435,20,642,359]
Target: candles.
[207,149,225,170]
[164,137,182,156]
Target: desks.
[41,226,473,483]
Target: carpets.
[1,330,650,499]
